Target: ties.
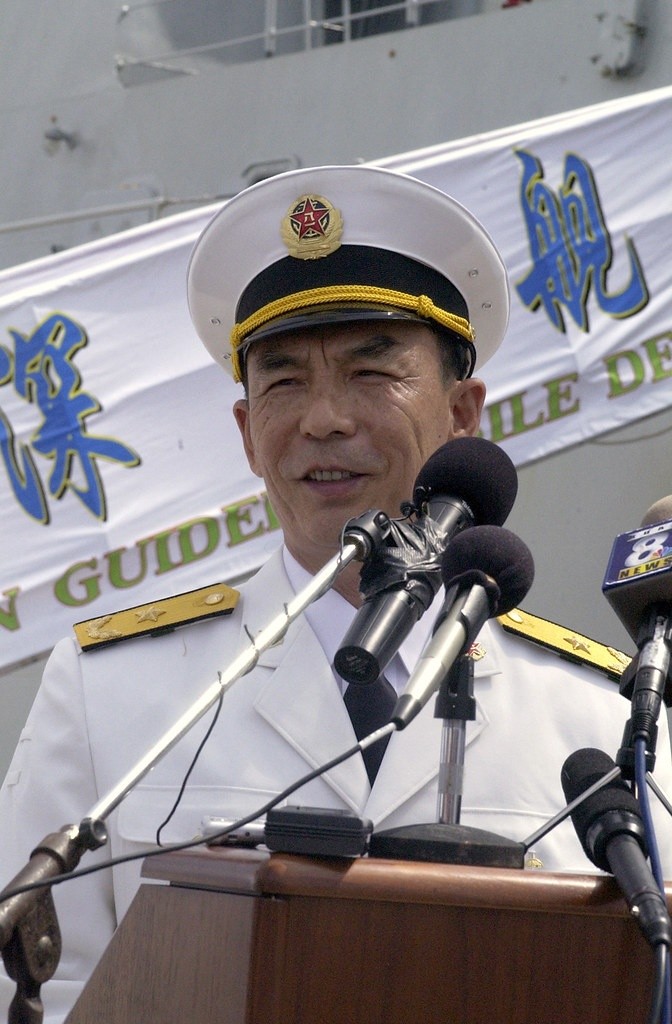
[343,671,399,789]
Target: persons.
[0,166,672,1024]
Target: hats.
[185,165,511,381]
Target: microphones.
[561,748,670,948]
[335,437,518,685]
[603,495,672,742]
[390,524,535,732]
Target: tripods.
[520,717,672,853]
